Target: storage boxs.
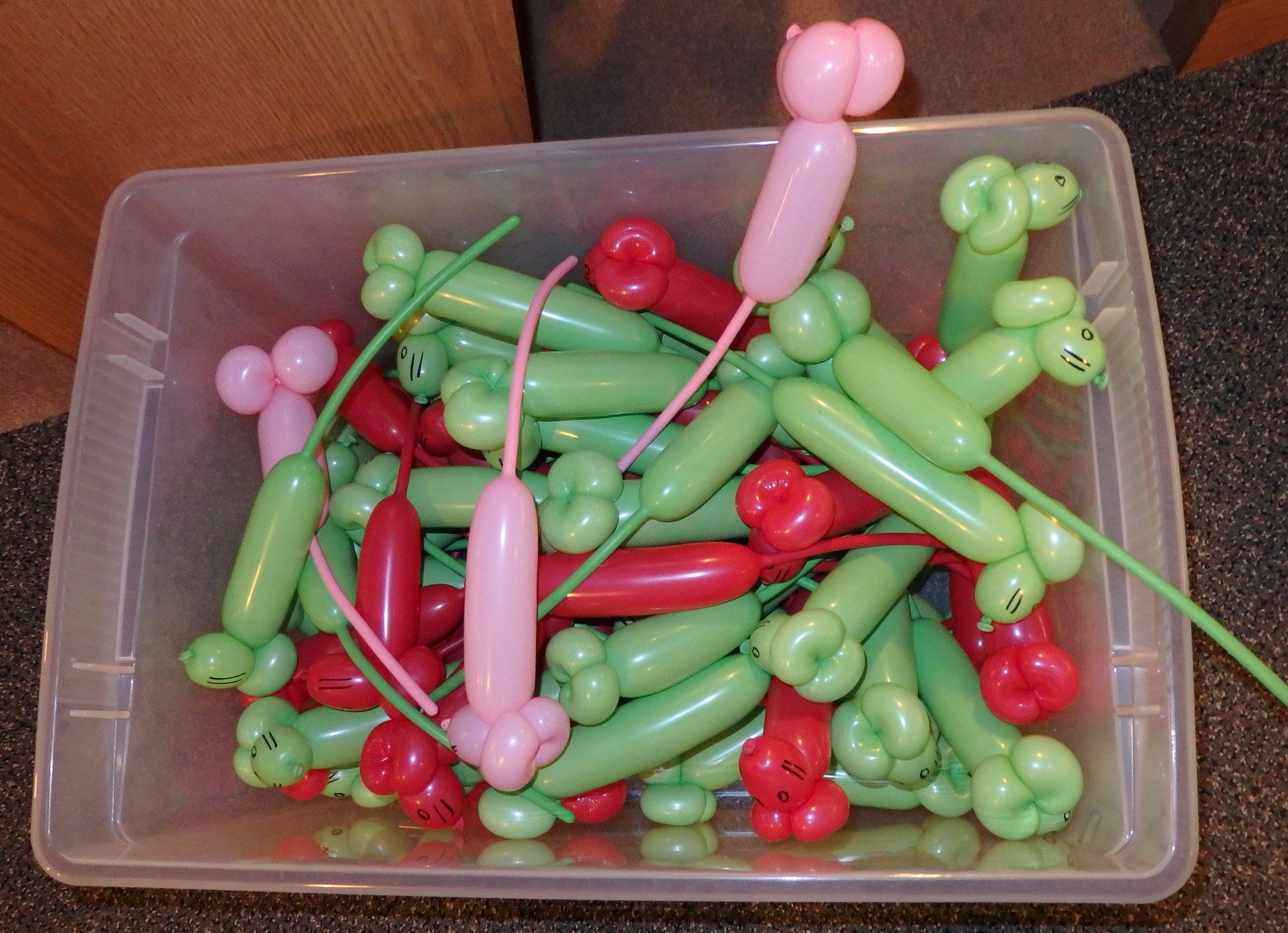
[29,104,1201,906]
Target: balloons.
[159,15,1287,871]
